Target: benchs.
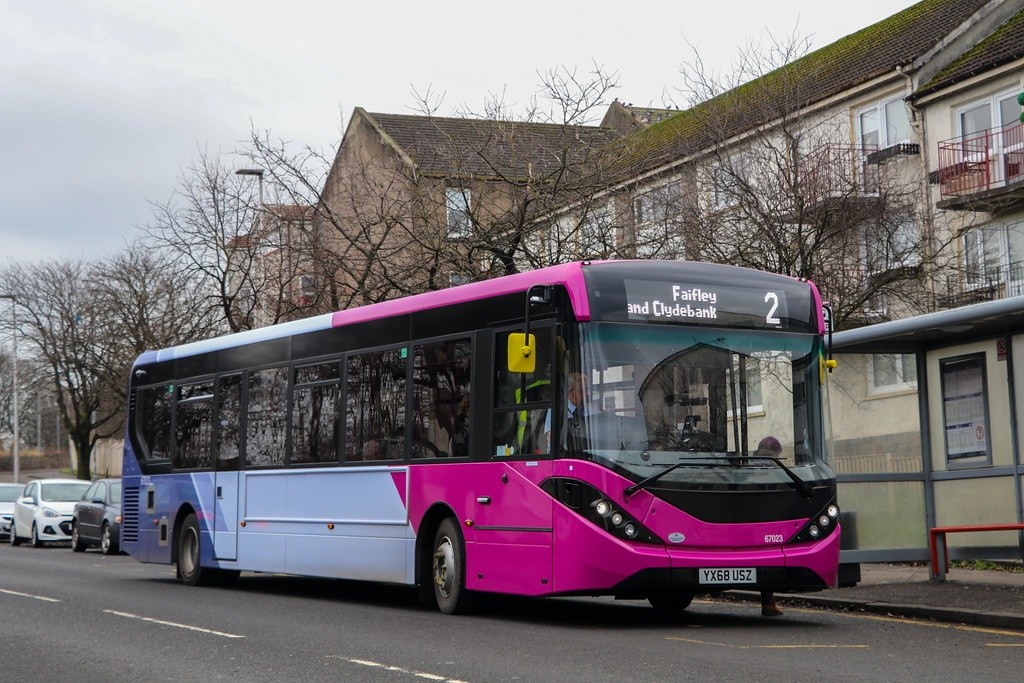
[929,522,1024,576]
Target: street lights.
[235,167,272,326]
[1,294,22,483]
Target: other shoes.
[762,603,783,616]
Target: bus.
[118,260,841,615]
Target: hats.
[759,437,782,454]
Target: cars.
[8,479,100,546]
[0,483,27,539]
[71,478,125,554]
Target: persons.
[362,440,383,460]
[759,590,785,616]
[752,437,782,459]
[544,372,602,454]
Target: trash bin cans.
[837,510,861,587]
